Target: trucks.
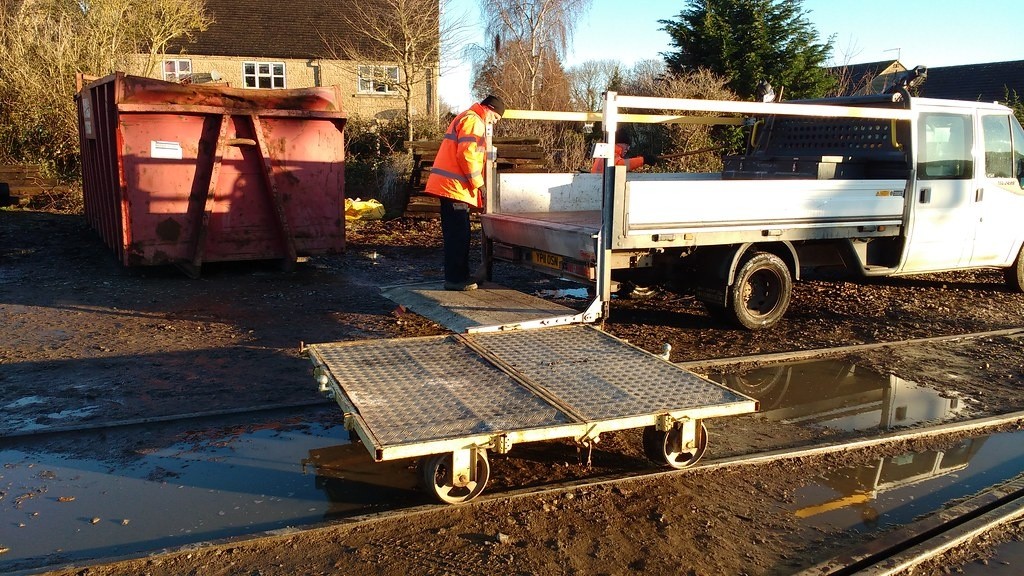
[477,93,1024,328]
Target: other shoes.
[463,275,483,285]
[444,280,478,290]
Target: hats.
[480,94,505,117]
[615,129,632,145]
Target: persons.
[426,94,505,291]
[590,131,658,173]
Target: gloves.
[478,184,486,199]
[643,152,658,166]
